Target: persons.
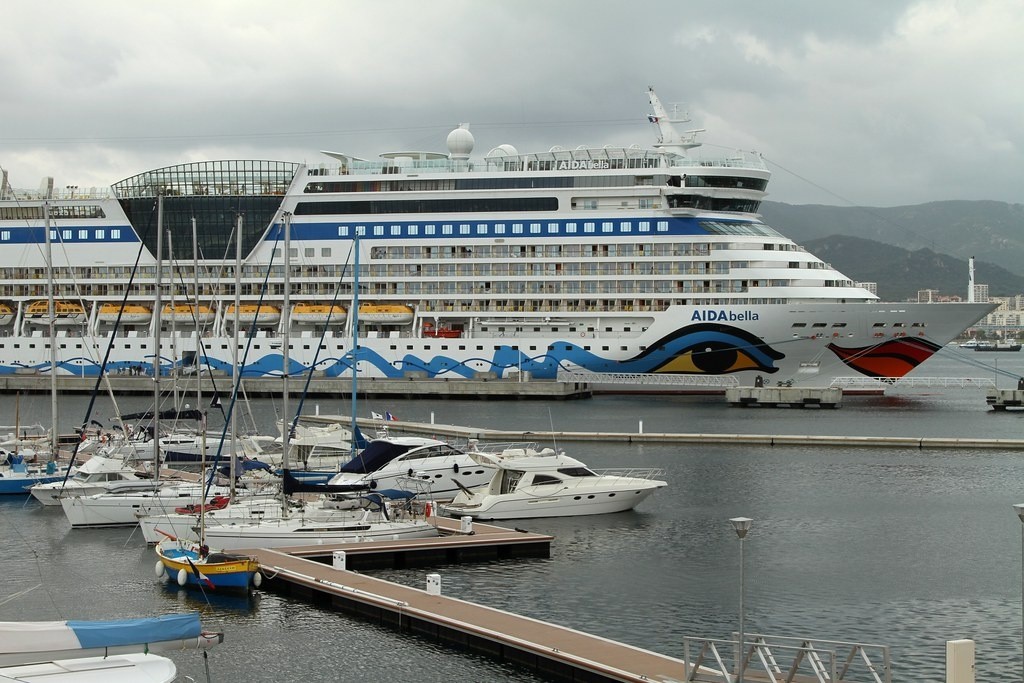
[199,542,209,557]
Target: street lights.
[730,514,757,683]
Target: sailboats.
[154,410,260,593]
[0,213,670,560]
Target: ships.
[0,87,1005,412]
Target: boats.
[224,302,281,325]
[423,322,463,339]
[986,377,1024,412]
[0,615,227,683]
[95,302,152,325]
[355,302,415,327]
[0,305,13,325]
[976,336,1022,353]
[164,579,258,615]
[289,301,350,327]
[160,303,216,325]
[959,336,992,350]
[26,299,87,327]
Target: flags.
[372,411,383,420]
[385,411,397,421]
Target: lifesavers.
[580,332,585,337]
[423,503,431,517]
[82,434,86,441]
